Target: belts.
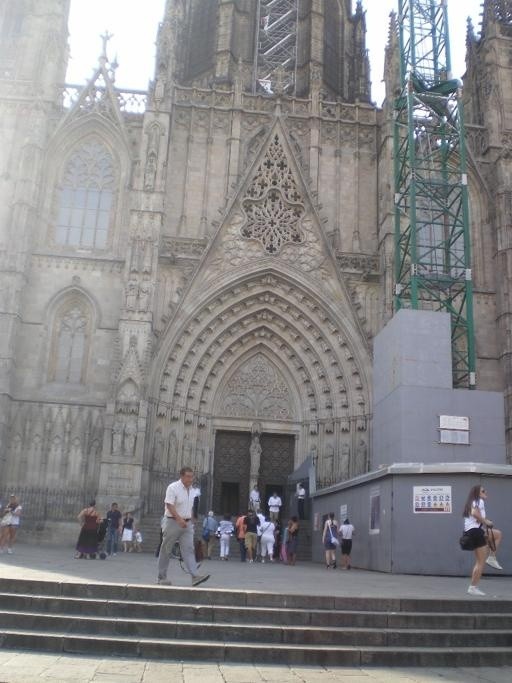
[167,516,191,521]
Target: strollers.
[84,519,111,560]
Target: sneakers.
[325,560,351,571]
[467,585,487,597]
[0,548,13,555]
[191,574,211,587]
[74,549,143,560]
[156,575,172,586]
[485,555,504,570]
[207,556,296,567]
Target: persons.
[0,493,23,555]
[73,499,143,560]
[156,466,211,586]
[322,511,339,569]
[463,483,503,596]
[190,476,306,566]
[339,518,356,571]
[155,509,181,560]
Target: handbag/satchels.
[202,516,210,542]
[459,522,487,551]
[257,536,261,542]
[331,536,340,547]
